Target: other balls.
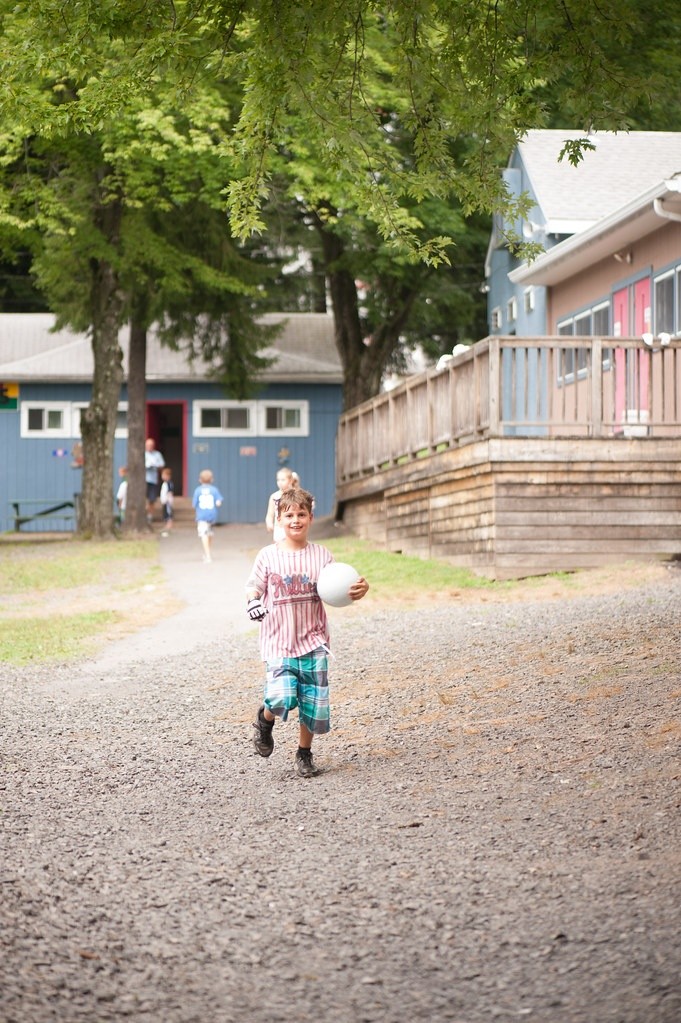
[317,563,360,607]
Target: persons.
[266,467,315,546]
[193,469,223,564]
[116,438,174,537]
[247,489,369,776]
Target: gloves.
[246,596,269,622]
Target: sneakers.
[294,750,319,778]
[252,705,275,757]
